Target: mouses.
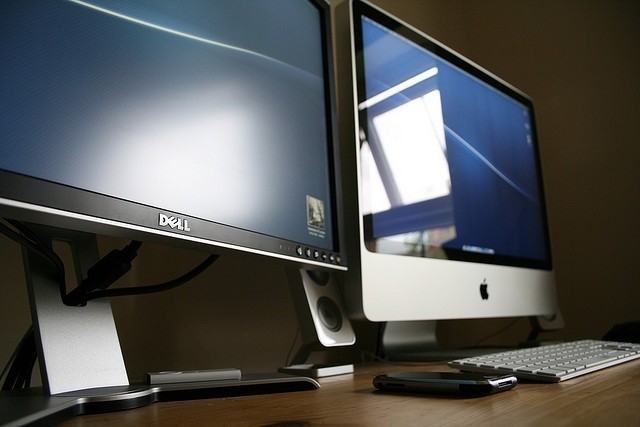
[601,321,639,345]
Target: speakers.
[519,306,566,347]
[277,263,355,378]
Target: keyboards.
[445,337,640,384]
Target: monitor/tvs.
[0,0,350,426]
[333,1,558,362]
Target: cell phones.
[372,371,518,399]
